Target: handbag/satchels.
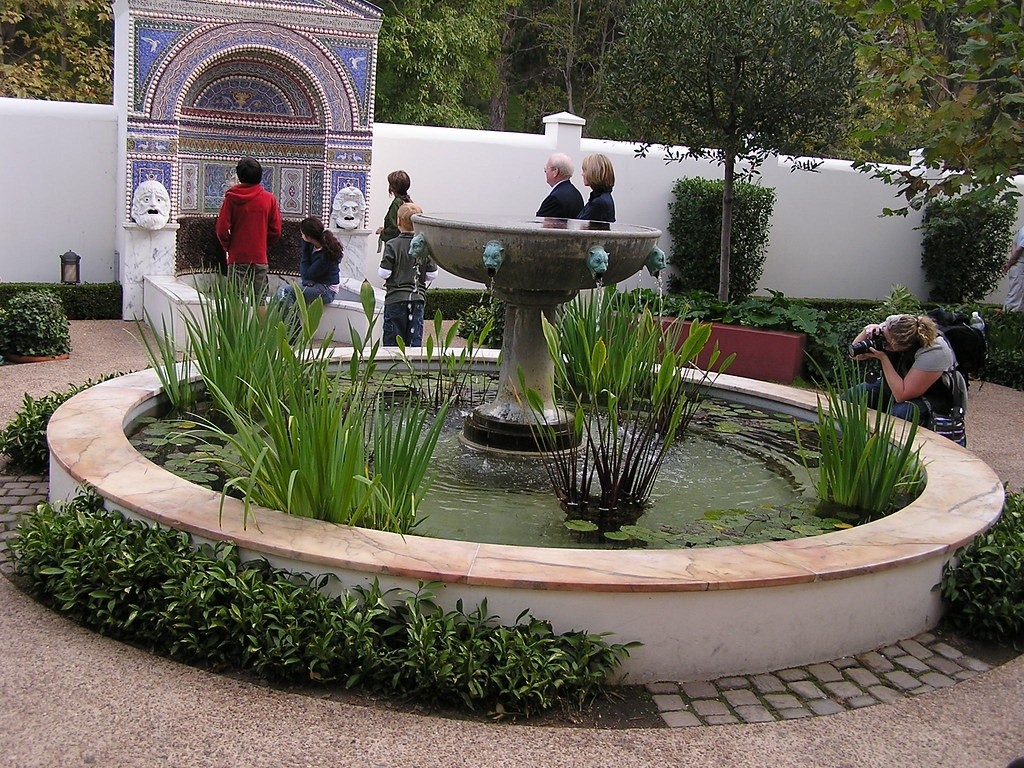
[929,412,967,448]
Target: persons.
[376,170,415,258]
[1001,226,1024,311]
[275,217,344,346]
[565,153,617,331]
[216,157,282,306]
[378,204,438,347]
[840,313,959,423]
[536,153,584,219]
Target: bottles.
[970,312,988,359]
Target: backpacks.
[926,308,989,374]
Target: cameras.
[847,328,889,358]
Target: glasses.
[544,165,554,172]
